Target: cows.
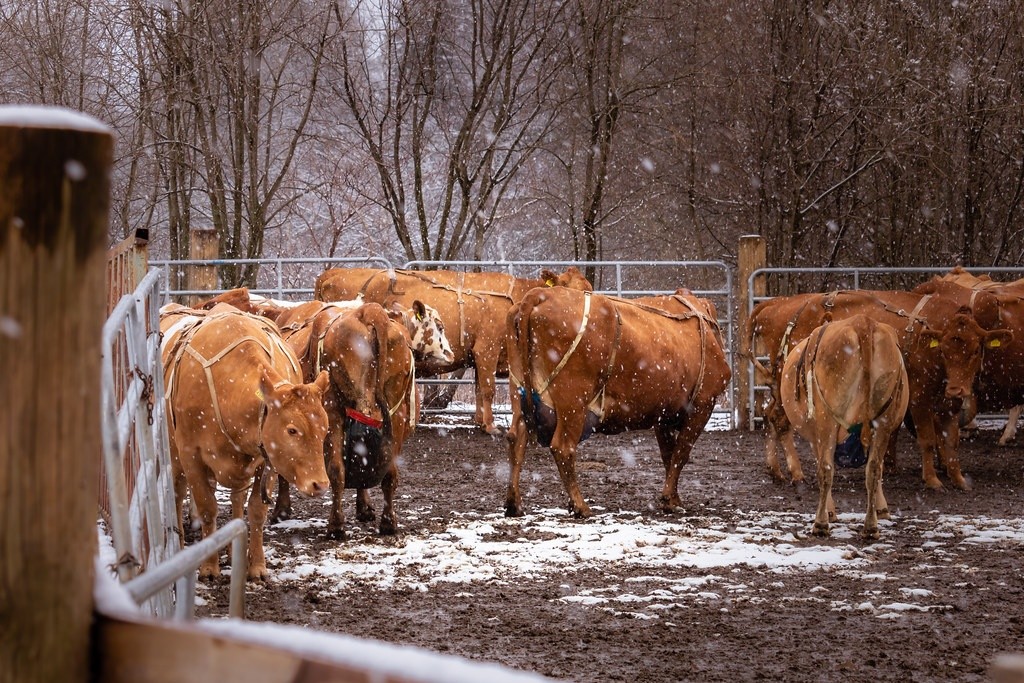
[158,265,1024,583]
